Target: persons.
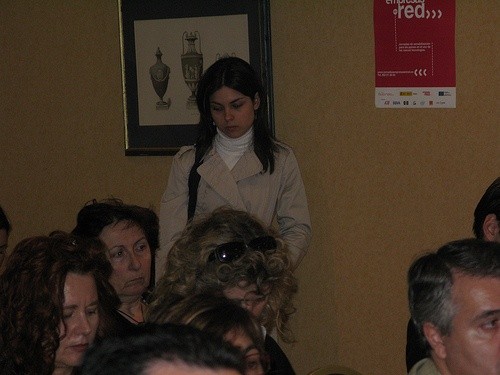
[82,322,249,375]
[405,177,500,372]
[0,205,11,265]
[69,199,160,333]
[0,232,122,375]
[156,291,270,375]
[162,208,298,347]
[405,238,500,375]
[153,57,312,288]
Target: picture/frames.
[117,0,275,156]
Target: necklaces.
[117,303,146,324]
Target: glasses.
[207,236,276,264]
